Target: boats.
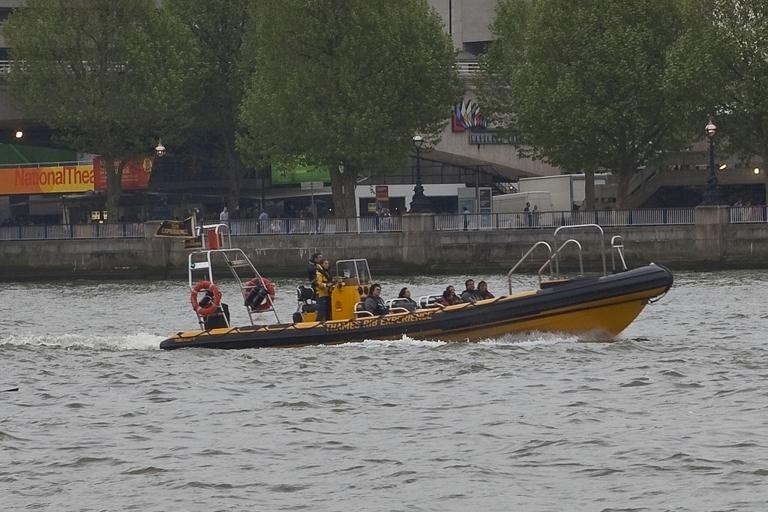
[159,222,673,352]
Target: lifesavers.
[191,281,222,315]
[246,278,275,310]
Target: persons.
[307,254,323,294]
[462,207,470,231]
[363,284,388,317]
[220,207,229,234]
[731,198,767,221]
[393,287,416,313]
[259,208,268,229]
[524,201,532,226]
[533,205,539,225]
[314,258,335,321]
[435,279,494,308]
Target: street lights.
[700,120,729,205]
[408,135,434,214]
[148,138,176,220]
